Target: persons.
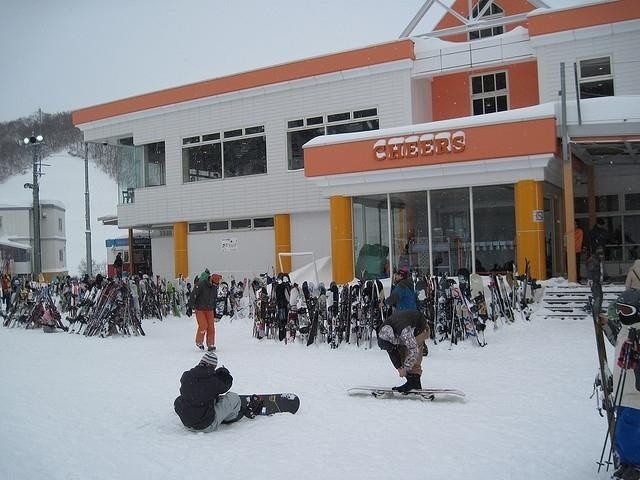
[0,270,22,312]
[174,350,263,433]
[383,263,429,356]
[186,274,222,351]
[377,309,430,392]
[625,246,640,290]
[588,218,621,284]
[575,220,583,283]
[597,291,640,480]
[198,267,210,281]
[114,252,123,281]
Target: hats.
[210,273,220,284]
[198,352,218,369]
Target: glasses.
[615,302,638,317]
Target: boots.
[398,375,425,392]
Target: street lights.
[18,130,44,283]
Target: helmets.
[616,286,640,312]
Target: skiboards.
[2,273,186,339]
[347,385,464,396]
[586,281,624,471]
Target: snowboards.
[236,393,300,416]
[230,273,384,350]
[414,257,536,348]
[214,282,228,318]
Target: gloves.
[186,308,192,317]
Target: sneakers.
[197,343,217,351]
[614,463,640,480]
